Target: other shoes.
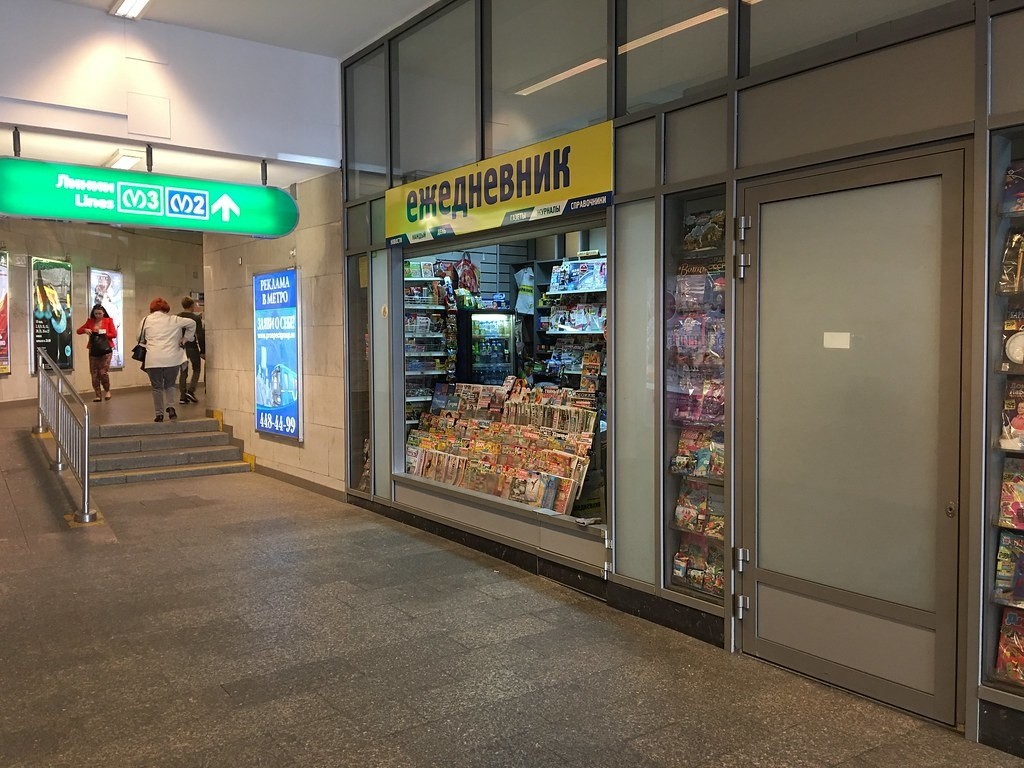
[105,391,111,400]
[154,415,163,422]
[168,407,177,419]
[93,396,102,402]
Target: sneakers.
[185,390,198,402]
[179,399,190,403]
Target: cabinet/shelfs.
[403,249,607,524]
[988,210,1023,697]
[671,253,724,600]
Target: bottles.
[471,319,510,383]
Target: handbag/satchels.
[131,317,146,361]
[90,332,112,357]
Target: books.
[405,376,597,517]
[580,350,601,392]
[548,260,607,371]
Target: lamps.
[103,149,145,169]
[108,0,154,22]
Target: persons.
[559,311,566,331]
[528,441,537,450]
[514,380,523,394]
[569,305,577,325]
[586,380,596,392]
[76,304,117,402]
[177,296,205,404]
[136,298,196,422]
